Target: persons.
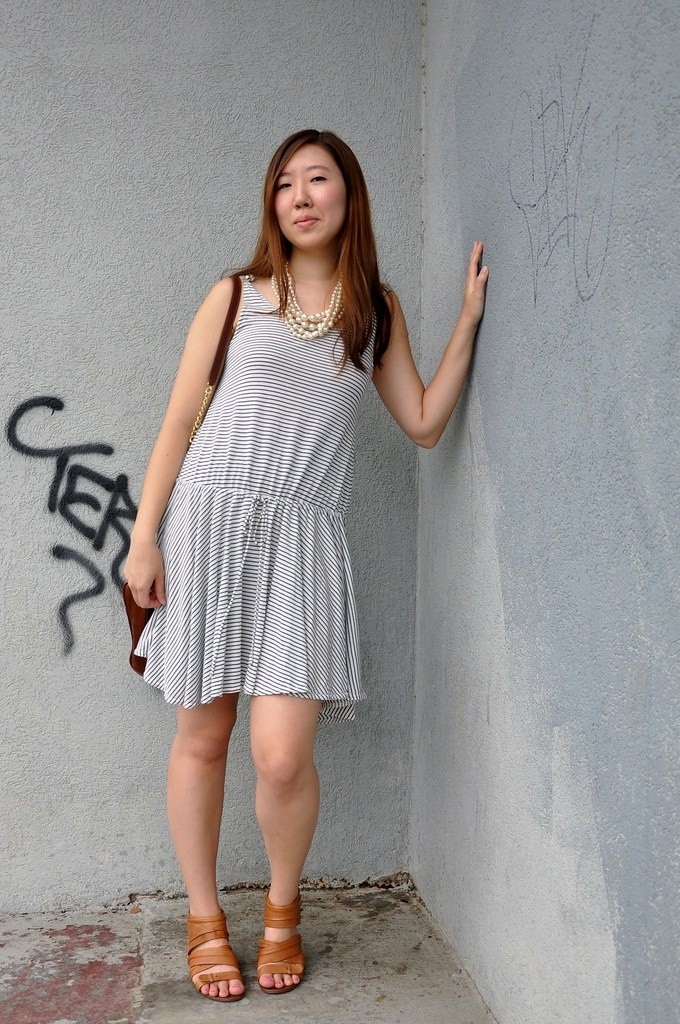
[125,128,489,1003]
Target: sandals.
[253,888,305,994]
[186,907,246,1003]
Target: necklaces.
[272,262,345,340]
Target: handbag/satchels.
[121,583,153,676]
